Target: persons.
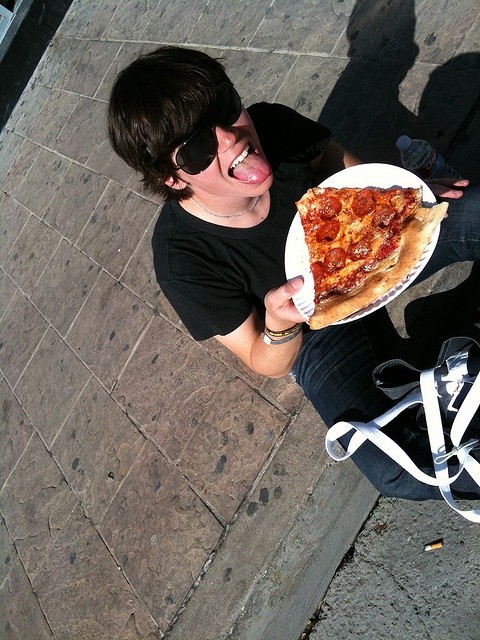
[108,45,478,503]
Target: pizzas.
[296,186,449,333]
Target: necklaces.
[190,195,262,219]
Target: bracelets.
[260,332,301,345]
[260,324,302,337]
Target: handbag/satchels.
[323,335,479,521]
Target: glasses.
[174,88,243,174]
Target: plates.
[284,160,442,329]
